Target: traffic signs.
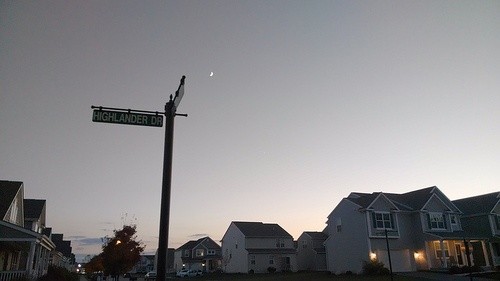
[92,110,164,128]
[170,77,186,118]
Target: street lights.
[182,263,185,271]
[202,263,205,273]
